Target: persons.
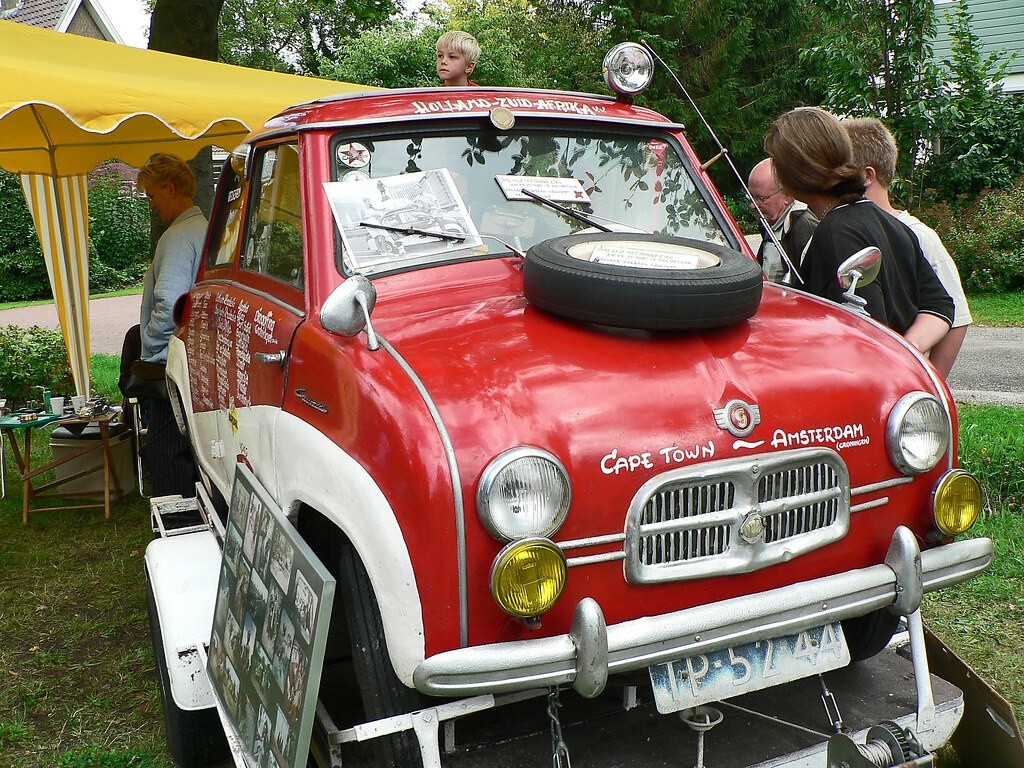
[210,490,313,768]
[360,196,392,256]
[410,183,454,249]
[377,180,388,198]
[746,107,973,651]
[136,153,210,523]
[436,31,482,86]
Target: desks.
[0,411,123,525]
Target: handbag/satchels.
[123,361,170,402]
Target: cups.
[71,395,85,414]
[50,397,64,416]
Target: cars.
[146,40,1024,768]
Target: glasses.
[147,183,176,201]
[746,188,783,204]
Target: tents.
[0,16,396,404]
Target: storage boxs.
[49,423,134,501]
